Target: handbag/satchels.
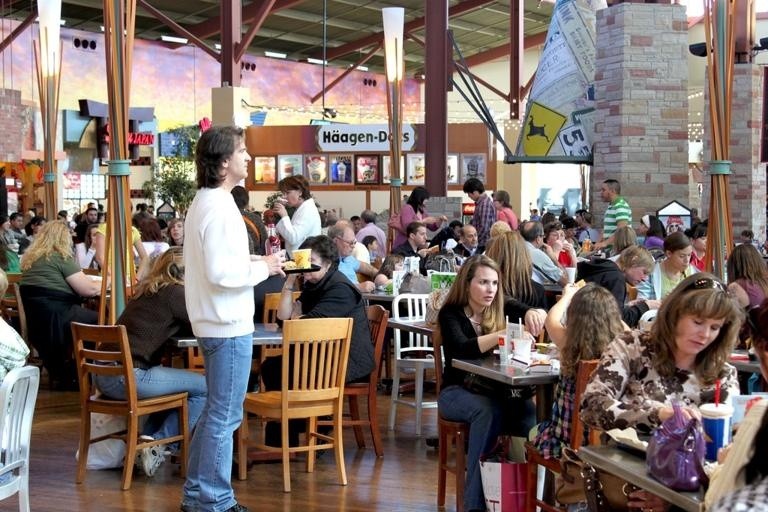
[425,255,464,327]
[82,417,127,469]
[646,413,705,492]
[479,451,527,512]
[398,272,431,294]
[556,457,641,510]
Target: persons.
[710,406,767,512]
[566,272,746,512]
[2,176,768,379]
[704,298,768,512]
[95,246,207,476]
[437,254,545,512]
[183,126,287,511]
[1,268,31,454]
[527,282,632,459]
[261,235,375,455]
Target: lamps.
[381,7,404,82]
[37,0,61,77]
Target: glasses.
[333,233,358,248]
[683,277,733,300]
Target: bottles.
[264,224,281,256]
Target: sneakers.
[316,416,333,458]
[122,434,166,477]
[180,503,251,512]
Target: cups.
[513,339,532,362]
[292,249,312,269]
[565,268,576,283]
[700,403,735,460]
